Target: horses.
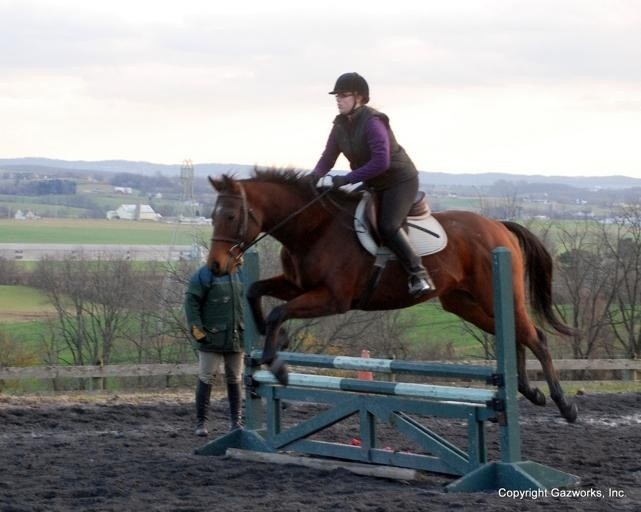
[206,164,582,424]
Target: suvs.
[104,202,159,223]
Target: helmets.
[329,73,369,103]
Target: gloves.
[332,175,350,189]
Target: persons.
[299,69,437,299]
[181,252,248,437]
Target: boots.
[387,228,436,296]
[196,378,212,436]
[227,383,243,430]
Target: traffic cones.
[354,346,375,394]
